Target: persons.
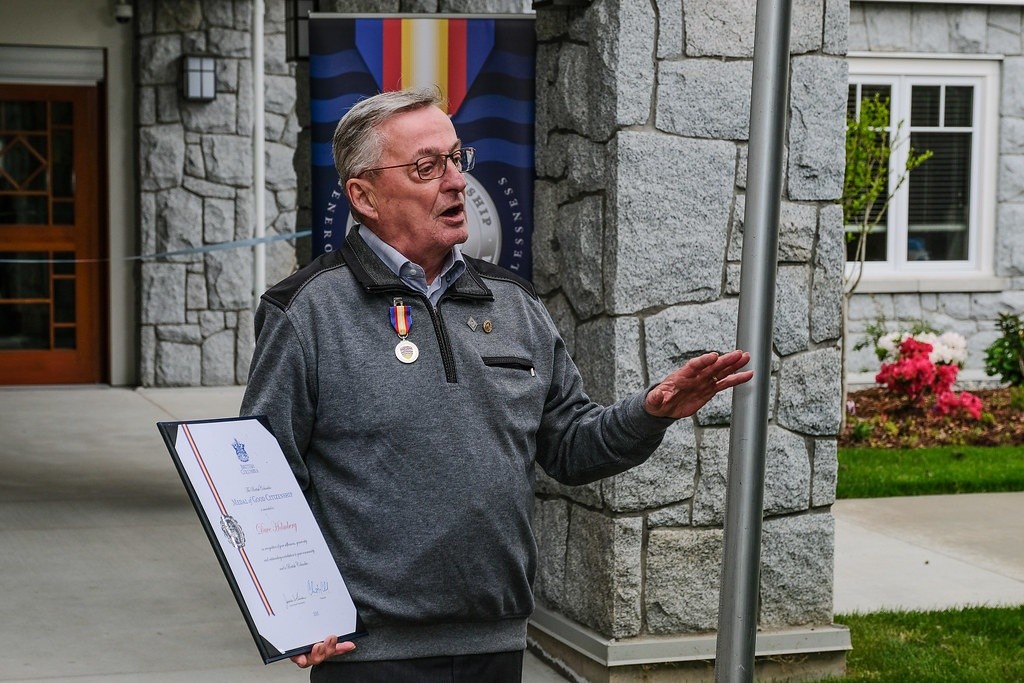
[244,84,753,683]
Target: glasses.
[358,147,476,180]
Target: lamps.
[179,52,223,105]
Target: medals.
[395,340,419,364]
